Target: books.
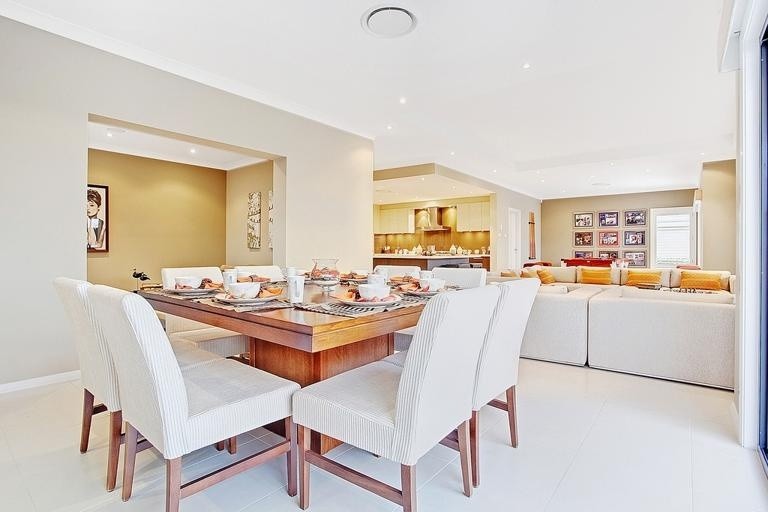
[636,282,663,290]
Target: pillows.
[501,266,729,291]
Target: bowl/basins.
[175,275,202,289]
[360,284,391,298]
[228,282,259,299]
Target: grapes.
[348,289,360,299]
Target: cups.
[287,275,305,302]
[286,267,296,276]
[222,270,238,291]
[368,275,385,284]
[418,271,446,291]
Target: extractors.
[414,207,450,232]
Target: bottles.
[383,243,491,256]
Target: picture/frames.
[570,208,648,268]
[87,184,110,254]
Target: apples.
[348,292,353,298]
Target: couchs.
[486,271,736,391]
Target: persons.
[87,189,105,249]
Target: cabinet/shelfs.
[373,209,415,234]
[455,201,489,232]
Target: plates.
[168,289,214,296]
[217,294,278,304]
[342,278,367,283]
[387,278,454,297]
[341,297,400,306]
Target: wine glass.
[310,259,344,293]
